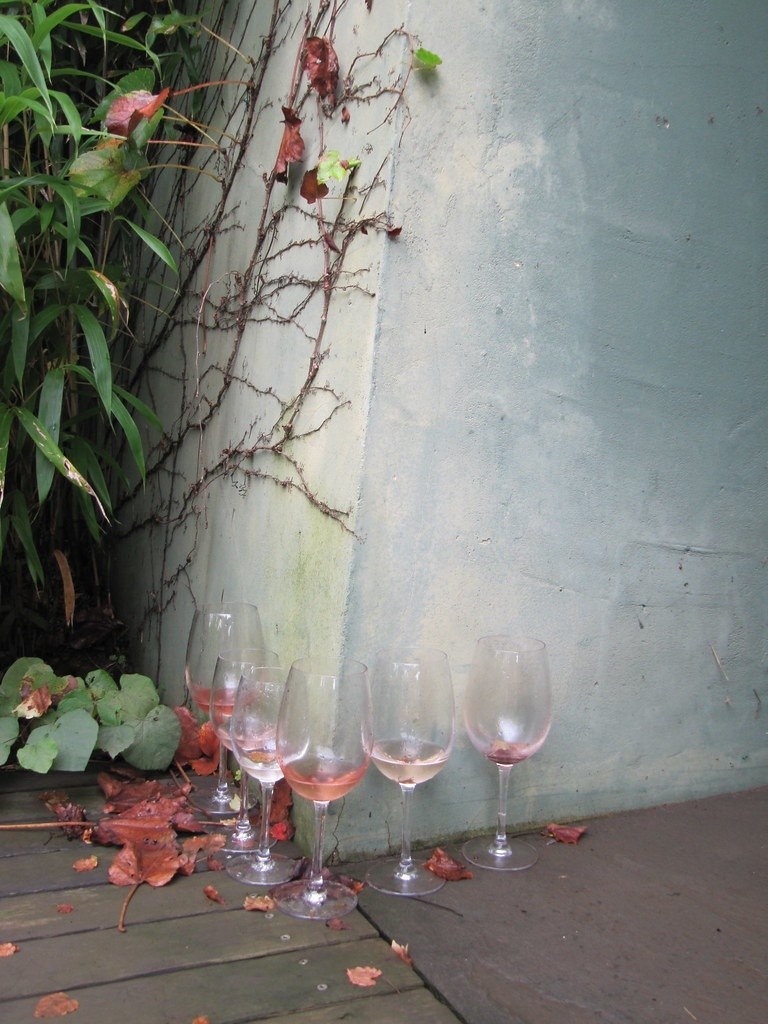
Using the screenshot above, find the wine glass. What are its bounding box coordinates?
[273,659,373,921]
[457,632,557,873]
[183,600,263,815]
[225,669,307,887]
[360,651,457,895]
[211,651,275,853]
